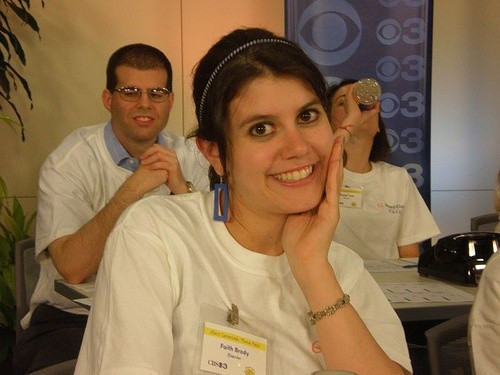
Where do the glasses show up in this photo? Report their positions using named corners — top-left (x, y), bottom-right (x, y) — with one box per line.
top-left (113, 86), bottom-right (171, 103)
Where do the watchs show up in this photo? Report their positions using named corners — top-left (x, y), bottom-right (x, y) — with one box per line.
top-left (170, 181), bottom-right (196, 195)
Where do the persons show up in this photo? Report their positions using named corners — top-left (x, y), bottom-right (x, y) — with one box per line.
top-left (20, 44), bottom-right (211, 375)
top-left (326, 78), bottom-right (441, 261)
top-left (75, 28), bottom-right (412, 375)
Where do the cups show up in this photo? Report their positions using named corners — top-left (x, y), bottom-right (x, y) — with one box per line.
top-left (352, 77), bottom-right (382, 110)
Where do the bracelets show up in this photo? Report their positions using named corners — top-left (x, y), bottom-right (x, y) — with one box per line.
top-left (338, 125), bottom-right (350, 134)
top-left (305, 293), bottom-right (351, 325)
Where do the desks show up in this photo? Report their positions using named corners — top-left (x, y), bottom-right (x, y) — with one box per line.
top-left (53, 257), bottom-right (475, 323)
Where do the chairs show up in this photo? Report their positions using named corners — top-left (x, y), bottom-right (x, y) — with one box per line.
top-left (424, 313), bottom-right (471, 375)
top-left (14, 238), bottom-right (35, 325)
